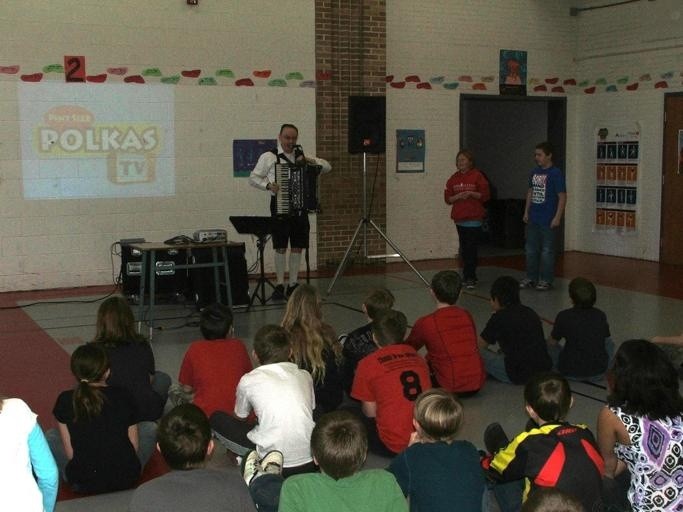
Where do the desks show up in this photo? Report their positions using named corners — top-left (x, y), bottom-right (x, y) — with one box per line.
top-left (129, 240), bottom-right (243, 341)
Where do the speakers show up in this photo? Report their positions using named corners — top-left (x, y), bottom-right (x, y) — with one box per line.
top-left (347, 94), bottom-right (387, 154)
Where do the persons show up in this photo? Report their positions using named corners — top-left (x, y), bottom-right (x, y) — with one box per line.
top-left (0, 272), bottom-right (683, 512)
top-left (518, 142), bottom-right (567, 292)
top-left (249, 124), bottom-right (332, 301)
top-left (444, 151), bottom-right (492, 285)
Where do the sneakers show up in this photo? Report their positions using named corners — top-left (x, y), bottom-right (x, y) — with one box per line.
top-left (238, 447), bottom-right (265, 486)
top-left (482, 421), bottom-right (510, 454)
top-left (519, 277), bottom-right (535, 290)
top-left (287, 283), bottom-right (300, 297)
top-left (272, 284), bottom-right (285, 301)
top-left (535, 280), bottom-right (553, 291)
top-left (259, 448), bottom-right (285, 476)
top-left (462, 274), bottom-right (479, 289)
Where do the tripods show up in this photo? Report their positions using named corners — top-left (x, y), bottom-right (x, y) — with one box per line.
top-left (327, 154), bottom-right (431, 299)
top-left (244, 243), bottom-right (280, 313)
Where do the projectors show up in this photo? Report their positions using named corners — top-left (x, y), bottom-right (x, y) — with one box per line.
top-left (193, 228), bottom-right (228, 242)
